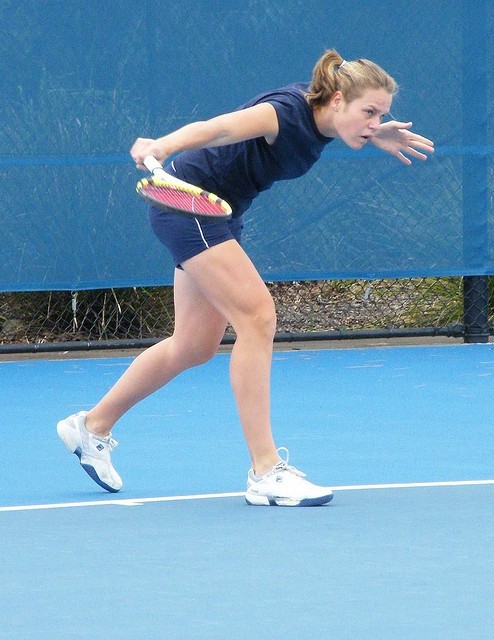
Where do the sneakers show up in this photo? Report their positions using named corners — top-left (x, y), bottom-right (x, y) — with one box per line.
top-left (56, 411), bottom-right (122, 492)
top-left (245, 447), bottom-right (334, 508)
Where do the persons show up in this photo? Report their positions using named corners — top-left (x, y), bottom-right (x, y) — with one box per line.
top-left (55, 47), bottom-right (435, 508)
